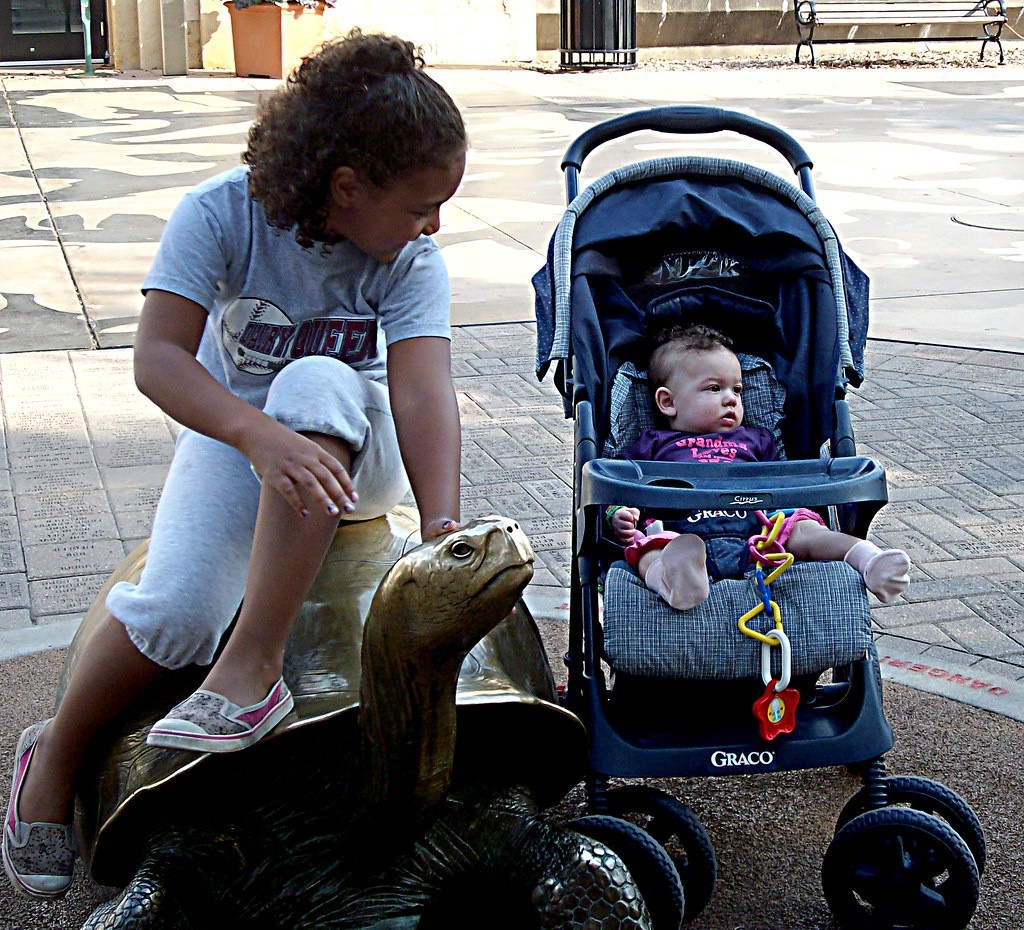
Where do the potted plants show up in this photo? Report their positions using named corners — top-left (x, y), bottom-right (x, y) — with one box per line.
top-left (224, 0), bottom-right (339, 80)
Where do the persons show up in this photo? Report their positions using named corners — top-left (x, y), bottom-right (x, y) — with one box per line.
top-left (603, 326), bottom-right (908, 609)
top-left (2, 33), bottom-right (468, 899)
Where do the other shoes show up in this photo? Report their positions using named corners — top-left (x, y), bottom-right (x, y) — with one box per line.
top-left (1, 717), bottom-right (75, 900)
top-left (146, 677), bottom-right (294, 754)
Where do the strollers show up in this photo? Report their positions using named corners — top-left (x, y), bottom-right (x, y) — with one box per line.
top-left (530, 102), bottom-right (990, 930)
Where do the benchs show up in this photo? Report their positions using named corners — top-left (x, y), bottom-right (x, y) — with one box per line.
top-left (794, 0), bottom-right (1009, 67)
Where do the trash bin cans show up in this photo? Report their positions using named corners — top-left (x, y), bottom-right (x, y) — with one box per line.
top-left (222, 1), bottom-right (338, 80)
top-left (559, 0), bottom-right (640, 71)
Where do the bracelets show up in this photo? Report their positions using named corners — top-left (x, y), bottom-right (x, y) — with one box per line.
top-left (606, 506), bottom-right (622, 524)
